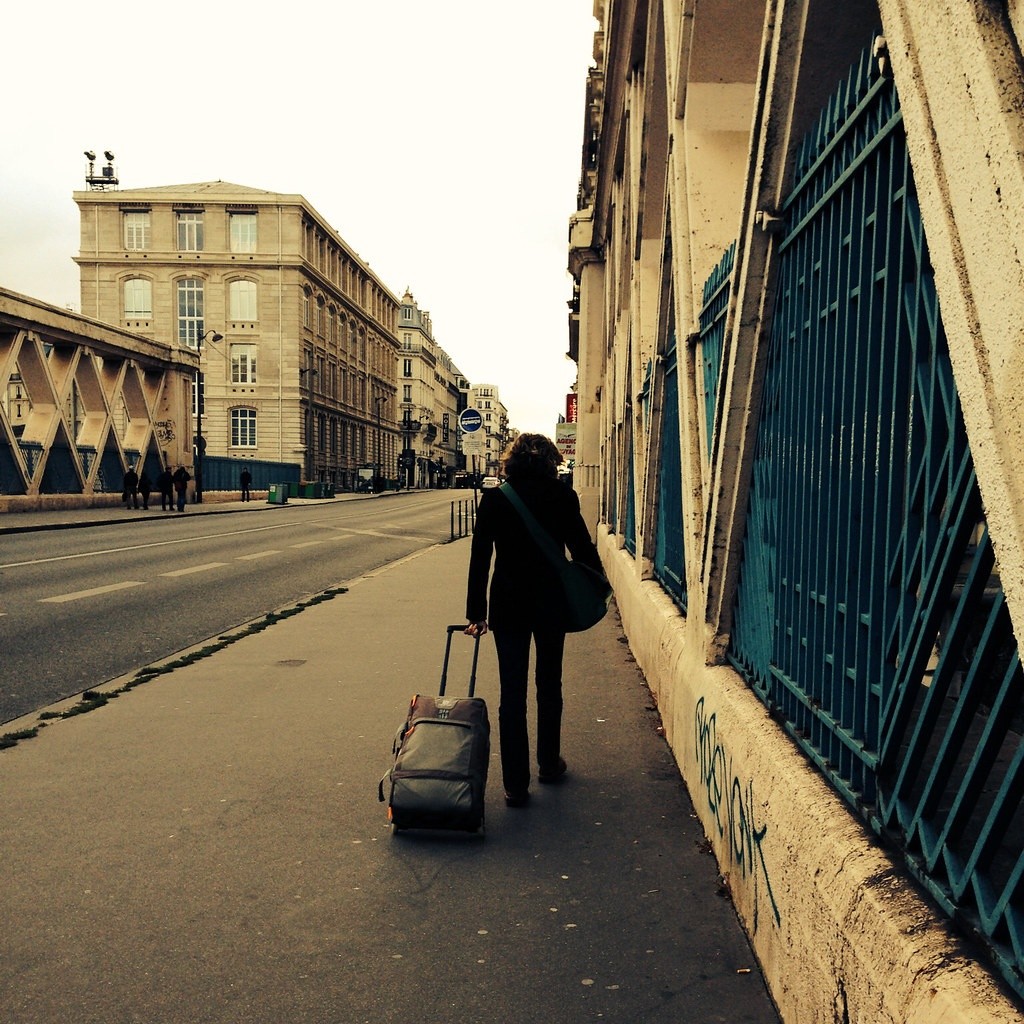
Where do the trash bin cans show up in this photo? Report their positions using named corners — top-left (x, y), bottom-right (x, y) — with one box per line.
top-left (266, 483), bottom-right (288, 504)
top-left (288, 482), bottom-right (298, 498)
top-left (298, 481), bottom-right (335, 498)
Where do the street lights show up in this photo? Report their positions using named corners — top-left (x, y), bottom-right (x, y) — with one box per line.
top-left (377, 397), bottom-right (388, 476)
top-left (195, 323), bottom-right (223, 503)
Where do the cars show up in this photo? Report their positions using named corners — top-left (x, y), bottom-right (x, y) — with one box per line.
top-left (481, 477), bottom-right (501, 491)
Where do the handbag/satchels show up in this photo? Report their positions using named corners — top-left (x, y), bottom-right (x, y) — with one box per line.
top-left (555, 560), bottom-right (609, 634)
top-left (122, 493), bottom-right (127, 502)
top-left (174, 481), bottom-right (183, 492)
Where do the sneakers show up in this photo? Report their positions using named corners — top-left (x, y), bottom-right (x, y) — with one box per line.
top-left (504, 786), bottom-right (531, 807)
top-left (537, 756), bottom-right (567, 784)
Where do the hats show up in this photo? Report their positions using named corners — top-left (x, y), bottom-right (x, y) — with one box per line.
top-left (129, 465), bottom-right (135, 470)
top-left (165, 466), bottom-right (172, 470)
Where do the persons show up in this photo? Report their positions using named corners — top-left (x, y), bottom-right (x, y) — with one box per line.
top-left (465, 435), bottom-right (613, 810)
top-left (122, 463), bottom-right (187, 513)
top-left (239, 467), bottom-right (252, 503)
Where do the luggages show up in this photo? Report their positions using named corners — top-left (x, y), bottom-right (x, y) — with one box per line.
top-left (378, 622), bottom-right (492, 841)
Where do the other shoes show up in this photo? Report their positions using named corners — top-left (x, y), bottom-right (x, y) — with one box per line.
top-left (247, 499), bottom-right (249, 502)
top-left (241, 499), bottom-right (244, 502)
